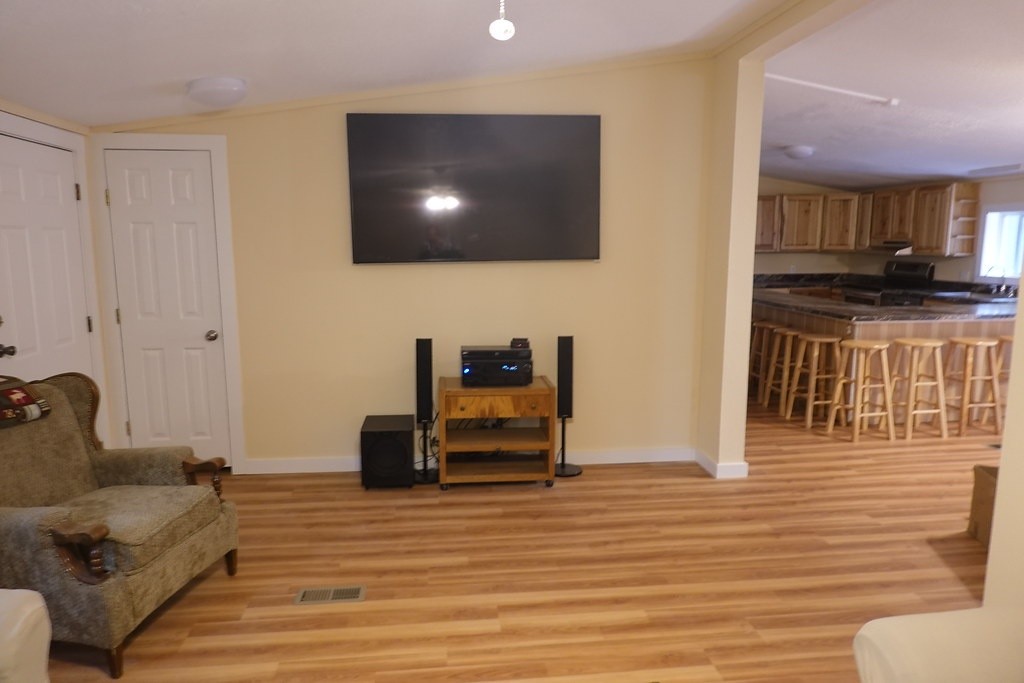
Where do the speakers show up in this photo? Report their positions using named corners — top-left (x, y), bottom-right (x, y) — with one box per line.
top-left (415, 338), bottom-right (440, 483)
top-left (360, 414), bottom-right (415, 490)
top-left (554, 337), bottom-right (582, 477)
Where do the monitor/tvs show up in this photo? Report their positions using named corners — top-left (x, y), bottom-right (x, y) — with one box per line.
top-left (347, 113), bottom-right (601, 264)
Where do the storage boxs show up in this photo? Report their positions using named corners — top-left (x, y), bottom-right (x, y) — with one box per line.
top-left (968, 463), bottom-right (998, 548)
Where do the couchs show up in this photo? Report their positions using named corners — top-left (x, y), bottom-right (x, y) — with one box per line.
top-left (0, 372), bottom-right (238, 683)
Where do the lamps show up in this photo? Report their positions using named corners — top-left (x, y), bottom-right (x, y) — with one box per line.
top-left (488, 0), bottom-right (515, 40)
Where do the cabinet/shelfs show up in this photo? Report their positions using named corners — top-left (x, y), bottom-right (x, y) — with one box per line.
top-left (438, 376), bottom-right (556, 491)
top-left (755, 179), bottom-right (980, 257)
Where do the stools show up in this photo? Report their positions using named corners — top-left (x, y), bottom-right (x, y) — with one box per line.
top-left (749, 320), bottom-right (784, 403)
top-left (786, 333), bottom-right (847, 429)
top-left (826, 339), bottom-right (897, 443)
top-left (981, 335), bottom-right (1013, 425)
top-left (764, 328), bottom-right (811, 417)
top-left (875, 337), bottom-right (950, 438)
top-left (930, 335), bottom-right (1002, 436)
